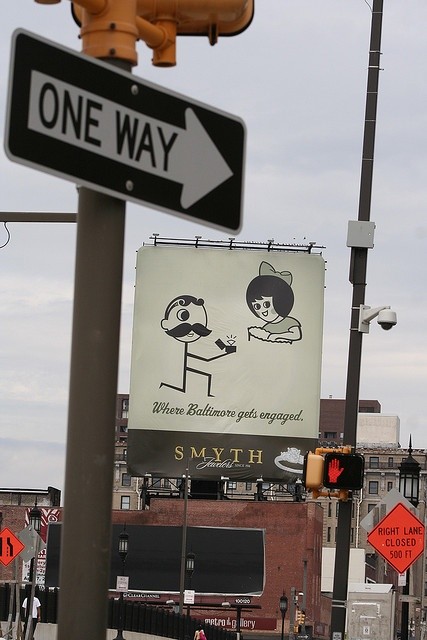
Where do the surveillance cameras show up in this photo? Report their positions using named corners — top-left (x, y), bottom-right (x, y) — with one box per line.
top-left (376, 309), bottom-right (400, 331)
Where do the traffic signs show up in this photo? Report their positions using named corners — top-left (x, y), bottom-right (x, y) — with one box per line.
top-left (5, 24), bottom-right (245, 239)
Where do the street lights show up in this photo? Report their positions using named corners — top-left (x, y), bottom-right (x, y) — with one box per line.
top-left (278, 588), bottom-right (288, 640)
top-left (112, 521), bottom-right (130, 639)
top-left (185, 544), bottom-right (195, 640)
top-left (26, 494), bottom-right (42, 640)
top-left (388, 435), bottom-right (422, 640)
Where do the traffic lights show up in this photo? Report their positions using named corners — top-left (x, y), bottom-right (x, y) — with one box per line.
top-left (321, 451), bottom-right (366, 490)
top-left (302, 450), bottom-right (324, 492)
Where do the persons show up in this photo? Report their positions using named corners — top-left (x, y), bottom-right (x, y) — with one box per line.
top-left (193, 625), bottom-right (207, 639)
top-left (22, 592), bottom-right (41, 640)
